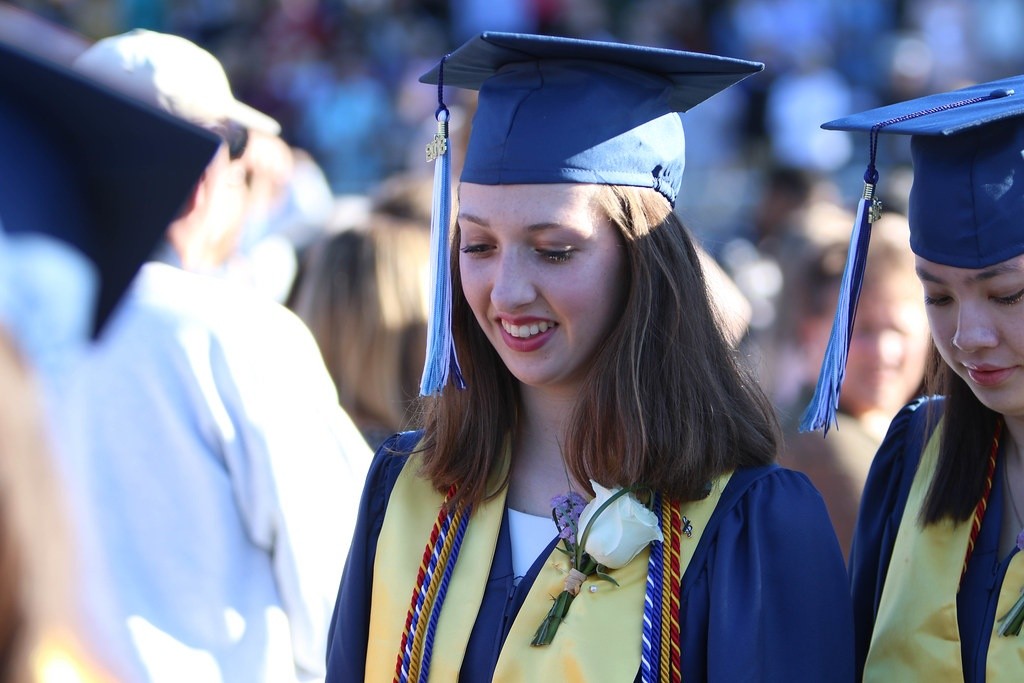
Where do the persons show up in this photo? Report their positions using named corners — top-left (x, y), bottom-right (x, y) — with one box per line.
top-left (324, 30), bottom-right (853, 683)
top-left (0, 1), bottom-right (1024, 683)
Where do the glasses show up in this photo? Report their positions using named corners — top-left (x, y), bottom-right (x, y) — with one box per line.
top-left (215, 122), bottom-right (247, 159)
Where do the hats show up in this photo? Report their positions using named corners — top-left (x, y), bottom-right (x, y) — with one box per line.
top-left (75, 27), bottom-right (281, 138)
top-left (1, 16), bottom-right (213, 341)
top-left (418, 31), bottom-right (765, 397)
top-left (795, 75), bottom-right (1024, 439)
